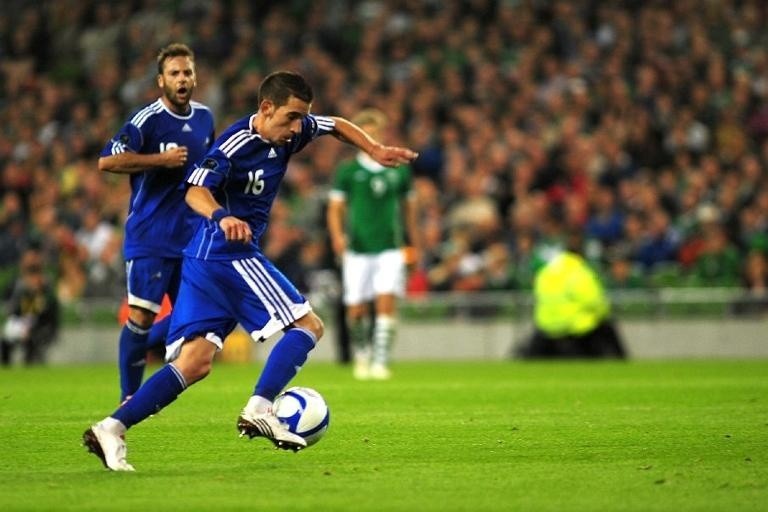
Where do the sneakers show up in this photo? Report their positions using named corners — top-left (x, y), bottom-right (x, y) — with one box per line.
top-left (236, 408), bottom-right (307, 453)
top-left (83, 422), bottom-right (136, 472)
top-left (353, 362), bottom-right (390, 380)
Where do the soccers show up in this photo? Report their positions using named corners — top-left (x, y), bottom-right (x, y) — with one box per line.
top-left (273, 386), bottom-right (330, 448)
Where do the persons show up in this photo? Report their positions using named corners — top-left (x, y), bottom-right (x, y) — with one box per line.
top-left (329, 109), bottom-right (418, 381)
top-left (1, 0), bottom-right (768, 368)
top-left (79, 43), bottom-right (420, 473)
top-left (98, 43), bottom-right (215, 417)
